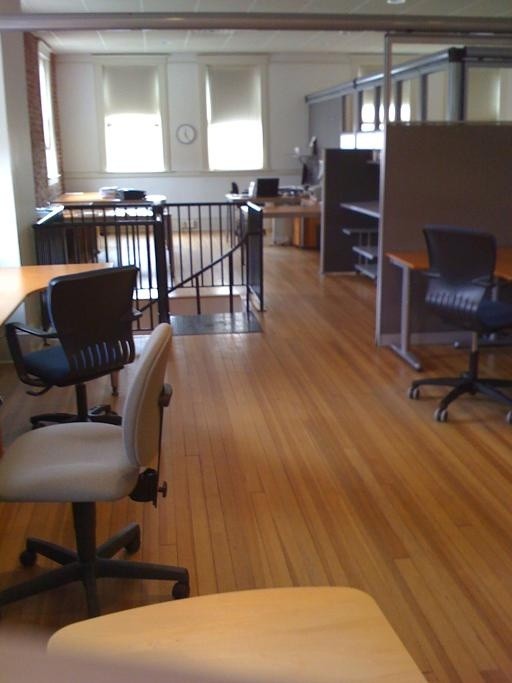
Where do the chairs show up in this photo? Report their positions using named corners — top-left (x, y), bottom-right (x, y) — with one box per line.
top-left (0, 322), bottom-right (191, 618)
top-left (47, 584), bottom-right (428, 683)
top-left (5, 265), bottom-right (139, 429)
top-left (408, 228), bottom-right (512, 425)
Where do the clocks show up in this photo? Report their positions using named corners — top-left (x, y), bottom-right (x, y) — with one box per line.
top-left (176, 124), bottom-right (196, 145)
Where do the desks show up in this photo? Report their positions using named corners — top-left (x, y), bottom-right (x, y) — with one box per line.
top-left (49, 192), bottom-right (175, 278)
top-left (226, 193), bottom-right (310, 248)
top-left (384, 245), bottom-right (512, 371)
top-left (240, 205), bottom-right (321, 249)
top-left (340, 201), bottom-right (380, 281)
top-left (0, 263), bottom-right (114, 328)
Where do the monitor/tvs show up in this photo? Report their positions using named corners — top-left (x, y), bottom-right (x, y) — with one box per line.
top-left (257, 178), bottom-right (279, 198)
